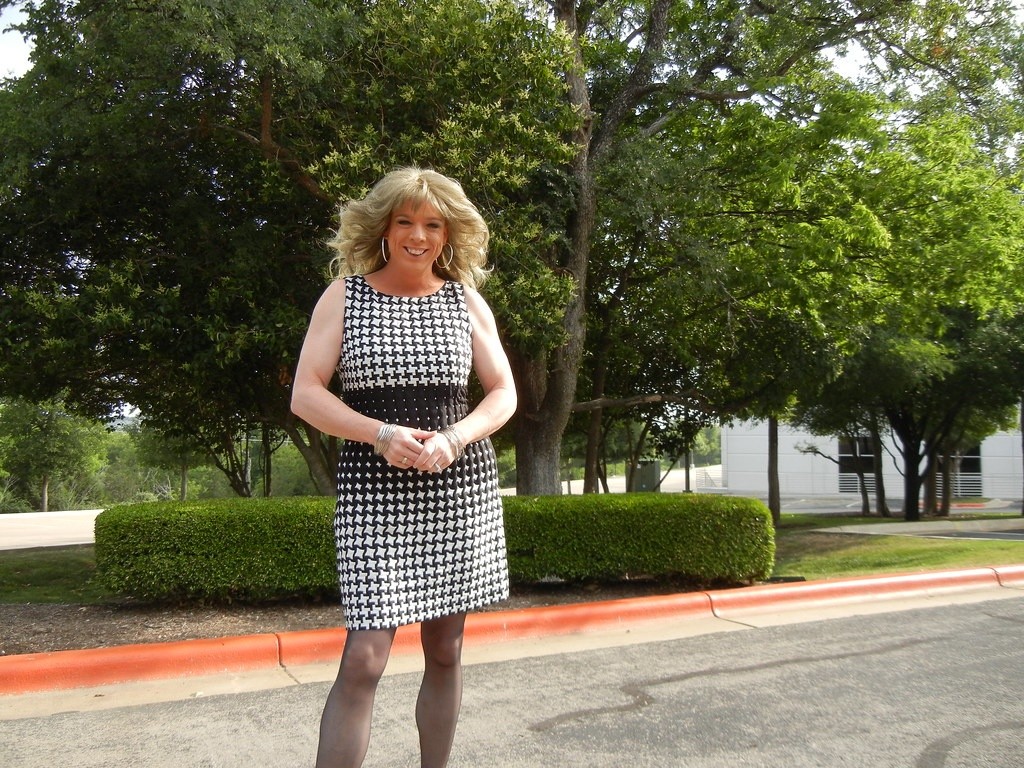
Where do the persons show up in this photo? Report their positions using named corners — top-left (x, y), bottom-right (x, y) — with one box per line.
top-left (290, 169), bottom-right (518, 767)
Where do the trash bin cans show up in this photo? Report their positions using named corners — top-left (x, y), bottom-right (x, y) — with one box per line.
top-left (625, 460), bottom-right (660, 493)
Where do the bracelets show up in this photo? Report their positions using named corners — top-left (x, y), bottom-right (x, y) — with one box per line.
top-left (374, 423), bottom-right (397, 457)
top-left (438, 426), bottom-right (464, 460)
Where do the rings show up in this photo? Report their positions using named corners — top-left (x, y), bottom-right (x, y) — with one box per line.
top-left (435, 463), bottom-right (441, 472)
top-left (402, 457), bottom-right (407, 464)
